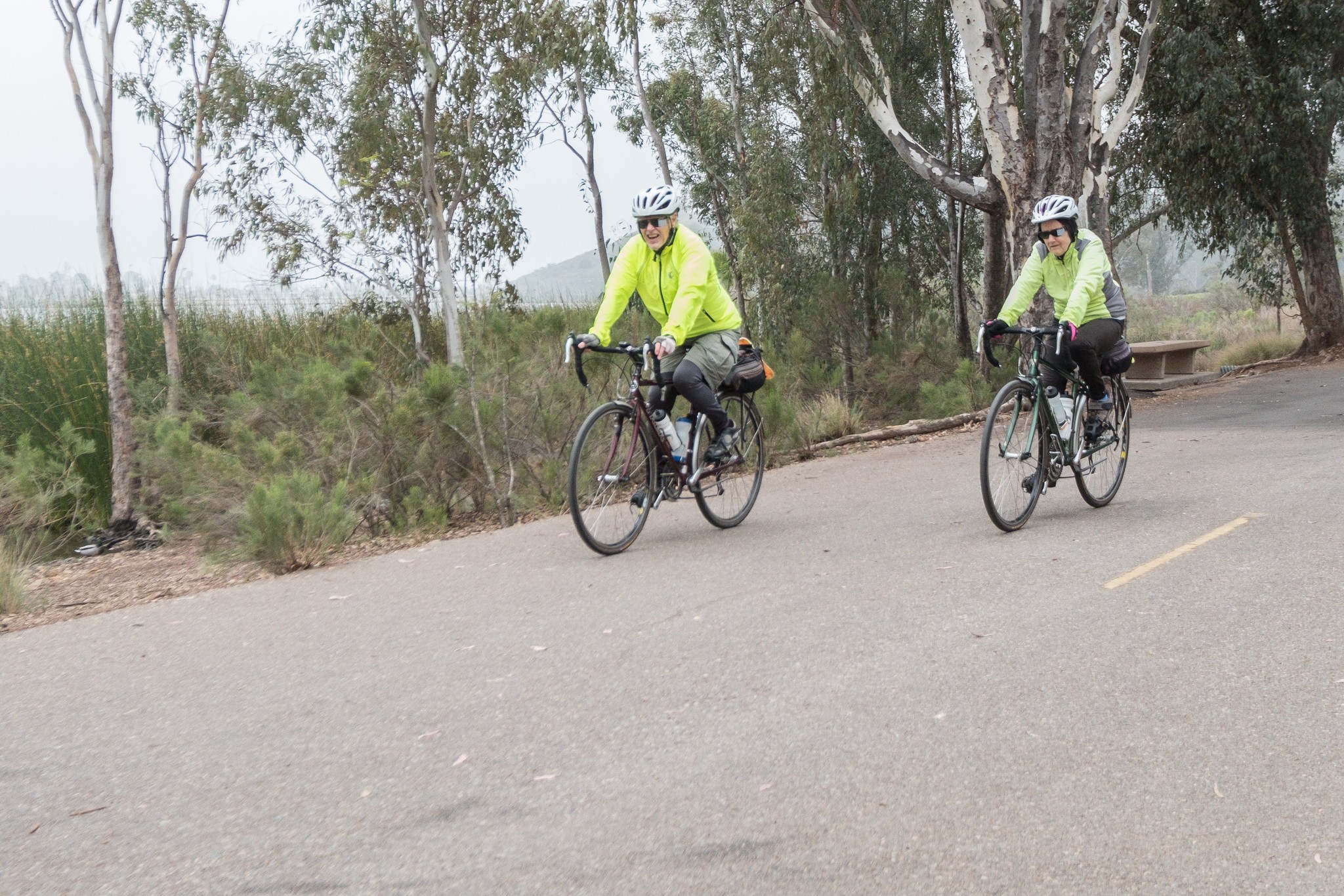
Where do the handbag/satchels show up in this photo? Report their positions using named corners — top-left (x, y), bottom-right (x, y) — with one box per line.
top-left (721, 347), bottom-right (767, 394)
top-left (1098, 334), bottom-right (1134, 376)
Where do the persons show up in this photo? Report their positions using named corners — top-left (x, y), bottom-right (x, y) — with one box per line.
top-left (986, 194), bottom-right (1127, 490)
top-left (574, 183), bottom-right (742, 508)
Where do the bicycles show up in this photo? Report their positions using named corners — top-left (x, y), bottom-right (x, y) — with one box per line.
top-left (979, 323), bottom-right (1132, 532)
top-left (568, 327), bottom-right (765, 555)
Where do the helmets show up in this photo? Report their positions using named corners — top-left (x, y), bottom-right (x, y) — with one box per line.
top-left (1030, 194), bottom-right (1079, 225)
top-left (631, 184), bottom-right (680, 218)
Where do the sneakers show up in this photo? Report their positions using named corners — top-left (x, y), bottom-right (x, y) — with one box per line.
top-left (703, 418), bottom-right (741, 463)
top-left (1020, 463), bottom-right (1057, 493)
top-left (629, 484), bottom-right (676, 507)
top-left (1083, 388), bottom-right (1113, 443)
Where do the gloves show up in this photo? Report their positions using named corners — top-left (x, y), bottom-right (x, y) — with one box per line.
top-left (1059, 320), bottom-right (1078, 344)
top-left (985, 318), bottom-right (1008, 341)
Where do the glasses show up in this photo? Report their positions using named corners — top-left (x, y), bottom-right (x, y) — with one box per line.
top-left (1037, 225), bottom-right (1067, 239)
top-left (637, 217), bottom-right (670, 230)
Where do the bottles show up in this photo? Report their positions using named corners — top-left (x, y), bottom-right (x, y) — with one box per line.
top-left (1045, 386), bottom-right (1068, 428)
top-left (1055, 390), bottom-right (1073, 441)
top-left (670, 413), bottom-right (693, 464)
top-left (653, 409), bottom-right (684, 451)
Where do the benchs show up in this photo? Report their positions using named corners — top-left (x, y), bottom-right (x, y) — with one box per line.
top-left (1123, 339), bottom-right (1211, 380)
